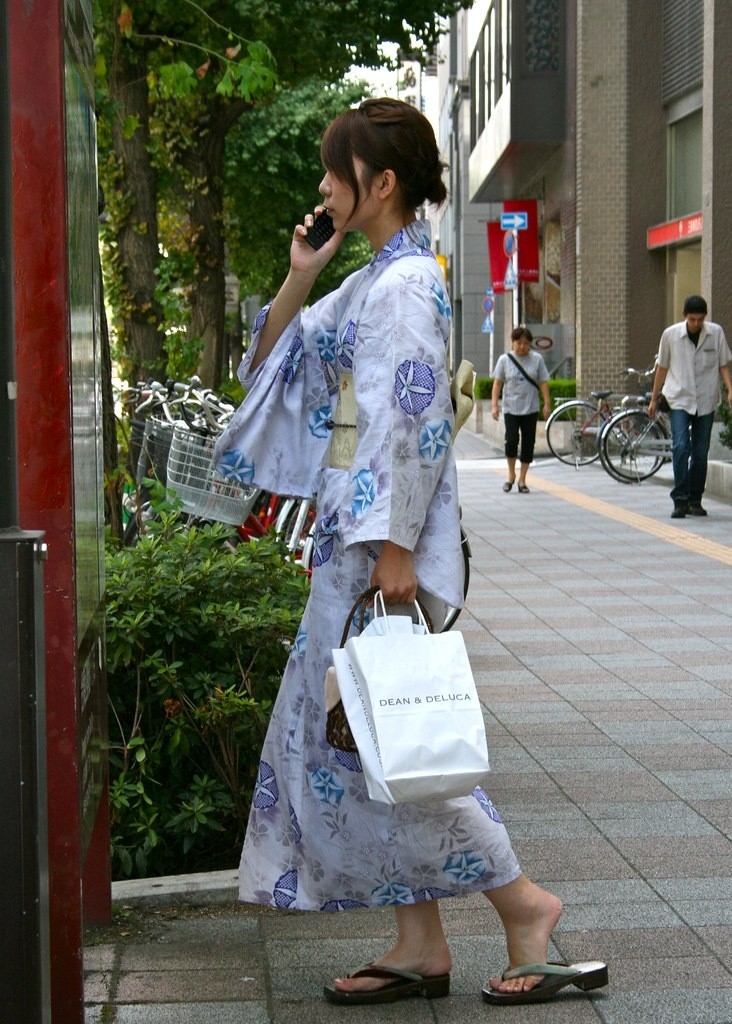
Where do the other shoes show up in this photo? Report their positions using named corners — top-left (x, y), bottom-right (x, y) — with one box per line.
top-left (688, 504), bottom-right (707, 516)
top-left (670, 501), bottom-right (687, 519)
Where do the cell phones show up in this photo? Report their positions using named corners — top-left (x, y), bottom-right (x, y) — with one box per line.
top-left (302, 208), bottom-right (336, 251)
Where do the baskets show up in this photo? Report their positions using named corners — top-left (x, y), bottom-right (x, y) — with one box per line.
top-left (136, 419), bottom-right (176, 498)
top-left (127, 419), bottom-right (145, 481)
top-left (166, 427), bottom-right (262, 525)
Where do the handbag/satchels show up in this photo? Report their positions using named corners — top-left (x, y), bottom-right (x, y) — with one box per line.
top-left (330, 591), bottom-right (493, 804)
top-left (325, 584), bottom-right (433, 754)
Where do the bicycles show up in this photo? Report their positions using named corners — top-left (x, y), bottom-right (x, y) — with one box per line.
top-left (544, 353), bottom-right (661, 472)
top-left (597, 393), bottom-right (692, 490)
top-left (123, 374), bottom-right (473, 633)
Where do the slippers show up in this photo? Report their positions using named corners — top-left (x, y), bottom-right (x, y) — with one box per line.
top-left (518, 483), bottom-right (529, 493)
top-left (325, 966), bottom-right (450, 1002)
top-left (503, 478), bottom-right (515, 492)
top-left (482, 960), bottom-right (608, 1003)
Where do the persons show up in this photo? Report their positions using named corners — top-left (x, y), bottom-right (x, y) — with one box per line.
top-left (210, 98), bottom-right (609, 1006)
top-left (647, 296), bottom-right (732, 519)
top-left (490, 327), bottom-right (553, 494)
